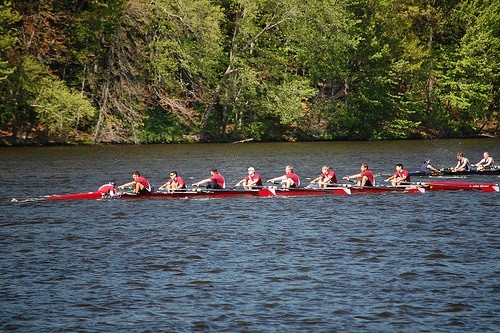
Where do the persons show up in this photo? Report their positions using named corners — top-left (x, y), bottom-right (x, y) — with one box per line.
top-left (475, 151), bottom-right (495, 172)
top-left (342, 162), bottom-right (375, 189)
top-left (192, 167), bottom-right (225, 191)
top-left (97, 181), bottom-right (123, 198)
top-left (448, 152), bottom-right (472, 173)
top-left (233, 167), bottom-right (262, 189)
top-left (266, 166), bottom-right (301, 191)
top-left (119, 170), bottom-right (152, 195)
top-left (158, 171), bottom-right (188, 192)
top-left (383, 164), bottom-right (411, 186)
top-left (421, 158), bottom-right (441, 176)
top-left (310, 166), bottom-right (338, 188)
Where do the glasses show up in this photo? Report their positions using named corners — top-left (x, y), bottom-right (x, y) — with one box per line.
top-left (133, 175), bottom-right (139, 178)
top-left (170, 173), bottom-right (175, 177)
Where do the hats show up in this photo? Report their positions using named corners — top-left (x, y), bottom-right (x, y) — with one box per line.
top-left (109, 180), bottom-right (118, 185)
top-left (322, 167), bottom-right (329, 170)
top-left (425, 159), bottom-right (432, 165)
top-left (286, 165), bottom-right (295, 170)
top-left (248, 167), bottom-right (255, 171)
top-left (209, 167), bottom-right (218, 172)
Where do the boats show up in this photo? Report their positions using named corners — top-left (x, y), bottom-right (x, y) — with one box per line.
top-left (380, 166), bottom-right (500, 177)
top-left (44, 182), bottom-right (499, 200)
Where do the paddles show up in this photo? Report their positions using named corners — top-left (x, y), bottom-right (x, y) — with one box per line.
top-left (118, 179), bottom-right (483, 196)
